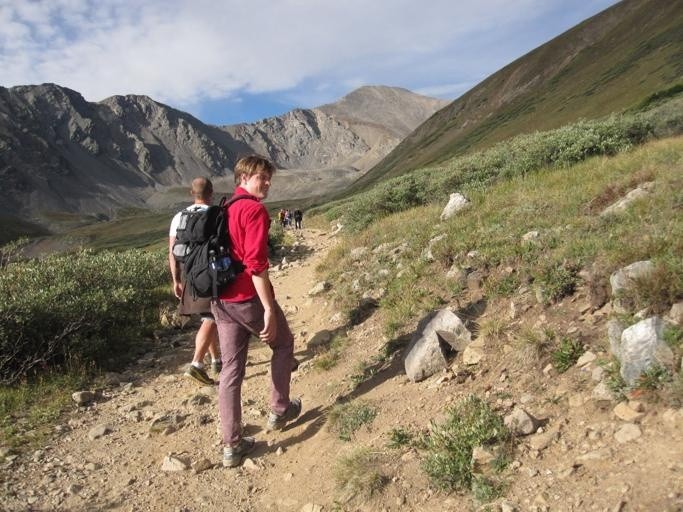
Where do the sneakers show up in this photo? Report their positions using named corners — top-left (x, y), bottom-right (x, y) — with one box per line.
top-left (211, 360), bottom-right (221, 375)
top-left (266, 397), bottom-right (302, 430)
top-left (184, 365), bottom-right (214, 387)
top-left (222, 436), bottom-right (255, 467)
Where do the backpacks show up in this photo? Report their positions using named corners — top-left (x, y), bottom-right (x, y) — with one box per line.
top-left (170, 208), bottom-right (208, 262)
top-left (181, 194), bottom-right (257, 307)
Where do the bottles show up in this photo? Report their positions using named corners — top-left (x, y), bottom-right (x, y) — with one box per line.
top-left (208, 246), bottom-right (235, 283)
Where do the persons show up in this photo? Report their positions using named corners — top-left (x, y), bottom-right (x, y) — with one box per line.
top-left (169, 177), bottom-right (222, 386)
top-left (279, 208), bottom-right (302, 230)
top-left (210, 155), bottom-right (302, 467)
